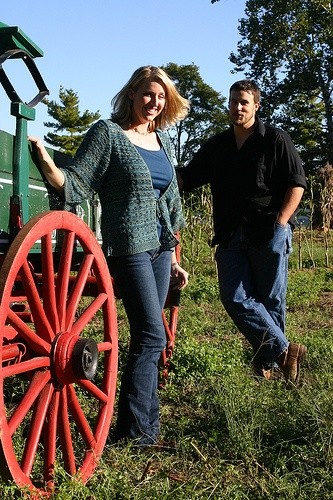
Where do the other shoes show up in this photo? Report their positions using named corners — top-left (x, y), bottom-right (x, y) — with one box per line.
top-left (136, 439), bottom-right (175, 457)
top-left (281, 343), bottom-right (307, 390)
top-left (252, 367), bottom-right (270, 386)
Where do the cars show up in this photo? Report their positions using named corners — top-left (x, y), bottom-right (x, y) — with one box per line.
top-left (287, 209), bottom-right (314, 227)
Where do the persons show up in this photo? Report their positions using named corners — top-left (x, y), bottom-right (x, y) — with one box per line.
top-left (26, 64), bottom-right (189, 447)
top-left (173, 80), bottom-right (307, 390)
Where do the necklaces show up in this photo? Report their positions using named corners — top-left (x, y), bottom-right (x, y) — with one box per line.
top-left (126, 122), bottom-right (150, 135)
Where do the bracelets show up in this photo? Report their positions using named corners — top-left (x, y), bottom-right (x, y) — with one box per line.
top-left (172, 262), bottom-right (177, 266)
